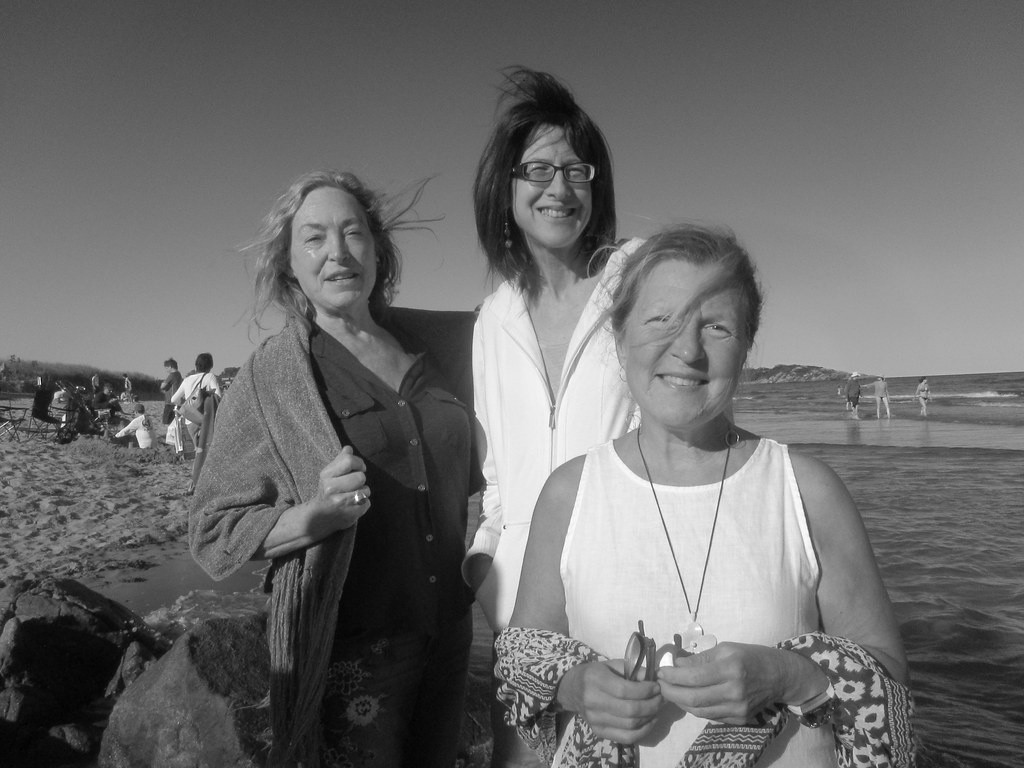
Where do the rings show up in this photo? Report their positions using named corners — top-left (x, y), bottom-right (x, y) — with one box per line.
top-left (354, 492), bottom-right (368, 506)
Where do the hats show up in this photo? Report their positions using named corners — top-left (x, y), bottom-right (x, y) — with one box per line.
top-left (134, 404), bottom-right (144, 410)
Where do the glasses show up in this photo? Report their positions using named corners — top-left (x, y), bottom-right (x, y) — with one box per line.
top-left (511, 161), bottom-right (596, 184)
top-left (623, 620), bottom-right (656, 681)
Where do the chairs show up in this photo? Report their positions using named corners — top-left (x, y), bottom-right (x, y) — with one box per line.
top-left (27, 370), bottom-right (73, 445)
top-left (0, 397), bottom-right (29, 444)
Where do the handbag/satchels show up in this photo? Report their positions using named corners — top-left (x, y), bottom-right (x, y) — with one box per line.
top-left (180, 372), bottom-right (208, 425)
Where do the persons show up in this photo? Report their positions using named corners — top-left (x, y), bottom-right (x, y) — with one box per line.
top-left (510, 223), bottom-right (917, 768)
top-left (470, 68), bottom-right (652, 768)
top-left (91, 354), bottom-right (224, 493)
top-left (193, 168), bottom-right (476, 766)
top-left (841, 372), bottom-right (933, 420)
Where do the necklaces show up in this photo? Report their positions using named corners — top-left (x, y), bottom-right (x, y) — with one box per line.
top-left (636, 413), bottom-right (733, 656)
